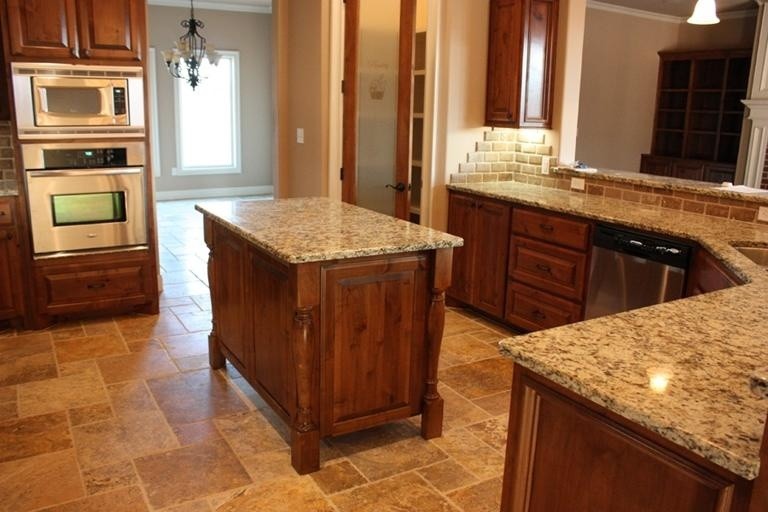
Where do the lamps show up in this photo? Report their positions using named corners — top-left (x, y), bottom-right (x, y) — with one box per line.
top-left (687, 0), bottom-right (721, 25)
top-left (162, 0), bottom-right (221, 90)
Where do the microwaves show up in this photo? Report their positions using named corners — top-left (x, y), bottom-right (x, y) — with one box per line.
top-left (9, 58), bottom-right (146, 137)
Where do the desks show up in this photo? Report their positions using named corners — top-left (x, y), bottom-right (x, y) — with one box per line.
top-left (195, 196), bottom-right (463, 474)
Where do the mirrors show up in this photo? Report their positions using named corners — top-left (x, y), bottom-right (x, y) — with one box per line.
top-left (559, 0), bottom-right (768, 188)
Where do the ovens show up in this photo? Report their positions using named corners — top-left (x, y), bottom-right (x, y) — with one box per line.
top-left (20, 142), bottom-right (155, 256)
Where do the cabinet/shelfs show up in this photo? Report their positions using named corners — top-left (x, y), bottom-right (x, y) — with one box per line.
top-left (640, 48), bottom-right (752, 184)
top-left (0, 1), bottom-right (146, 66)
top-left (31, 249), bottom-right (159, 330)
top-left (448, 191), bottom-right (592, 333)
top-left (0, 195), bottom-right (31, 329)
top-left (686, 249), bottom-right (744, 301)
top-left (485, 0), bottom-right (560, 128)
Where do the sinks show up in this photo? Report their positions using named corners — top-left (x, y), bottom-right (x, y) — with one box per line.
top-left (728, 240), bottom-right (768, 267)
